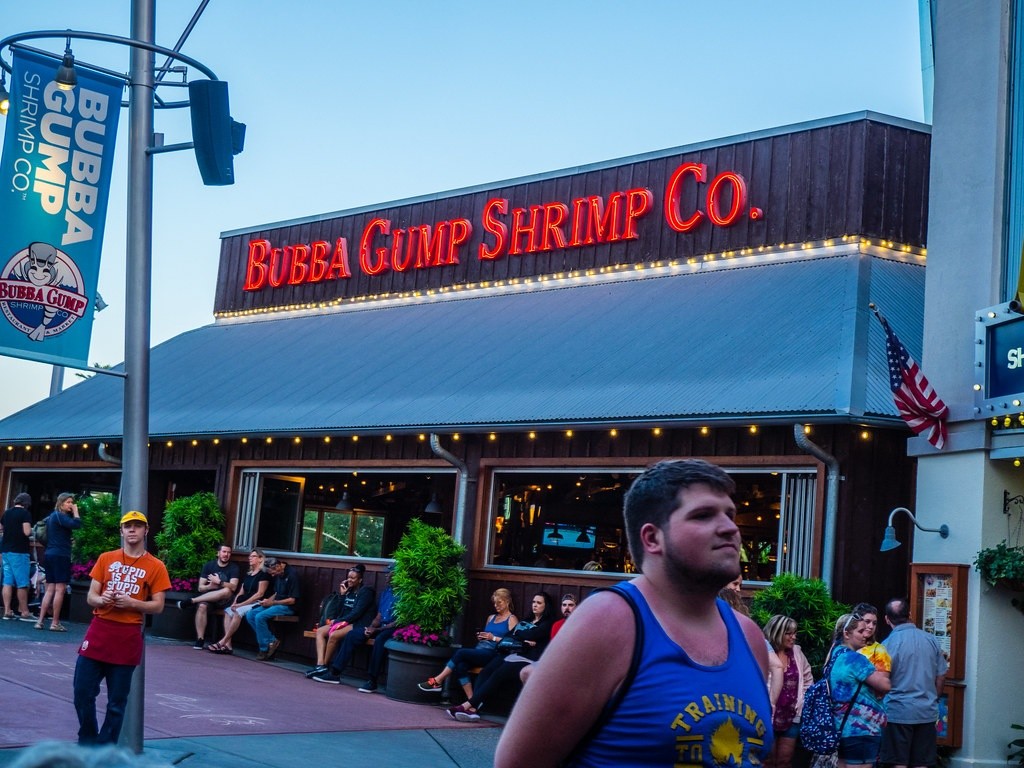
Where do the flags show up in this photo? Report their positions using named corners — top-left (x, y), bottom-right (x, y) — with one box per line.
top-left (882, 317), bottom-right (950, 450)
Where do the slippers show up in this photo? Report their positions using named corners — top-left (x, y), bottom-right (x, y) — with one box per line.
top-left (207, 642), bottom-right (221, 650)
top-left (214, 645), bottom-right (233, 654)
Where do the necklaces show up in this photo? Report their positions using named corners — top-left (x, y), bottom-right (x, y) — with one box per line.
top-left (122, 546), bottom-right (146, 573)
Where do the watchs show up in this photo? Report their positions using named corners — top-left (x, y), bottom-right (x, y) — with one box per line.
top-left (219, 579), bottom-right (224, 585)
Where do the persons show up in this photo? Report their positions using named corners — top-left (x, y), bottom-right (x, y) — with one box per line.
top-left (73, 510), bottom-right (173, 746)
top-left (493, 457), bottom-right (772, 768)
top-left (32, 492), bottom-right (81, 631)
top-left (418, 587), bottom-right (577, 722)
top-left (176, 543), bottom-right (397, 695)
top-left (720, 572), bottom-right (947, 768)
top-left (0, 493), bottom-right (39, 623)
top-left (245, 558), bottom-right (302, 661)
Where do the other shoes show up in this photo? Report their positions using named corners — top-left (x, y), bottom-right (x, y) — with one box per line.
top-left (456, 711), bottom-right (480, 722)
top-left (257, 652), bottom-right (268, 660)
top-left (267, 639), bottom-right (281, 658)
top-left (305, 665), bottom-right (328, 677)
top-left (446, 705), bottom-right (464, 720)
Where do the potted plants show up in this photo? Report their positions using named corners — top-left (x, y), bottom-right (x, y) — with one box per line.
top-left (973, 539), bottom-right (1024, 594)
top-left (384, 517), bottom-right (470, 708)
top-left (151, 491), bottom-right (226, 642)
top-left (66, 494), bottom-right (122, 622)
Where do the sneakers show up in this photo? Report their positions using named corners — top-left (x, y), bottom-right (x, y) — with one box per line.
top-left (20, 613), bottom-right (38, 622)
top-left (3, 611), bottom-right (17, 620)
top-left (312, 672), bottom-right (340, 684)
top-left (178, 598), bottom-right (192, 609)
top-left (359, 680), bottom-right (377, 692)
top-left (417, 677), bottom-right (442, 692)
top-left (194, 638), bottom-right (204, 650)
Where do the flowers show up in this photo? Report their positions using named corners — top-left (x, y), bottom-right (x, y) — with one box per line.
top-left (167, 577), bottom-right (201, 593)
top-left (70, 559), bottom-right (97, 580)
top-left (392, 623), bottom-right (453, 647)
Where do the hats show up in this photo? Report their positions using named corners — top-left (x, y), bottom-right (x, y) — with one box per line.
top-left (120, 511), bottom-right (147, 527)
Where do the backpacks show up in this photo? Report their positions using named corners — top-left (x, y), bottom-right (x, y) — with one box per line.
top-left (799, 647), bottom-right (862, 754)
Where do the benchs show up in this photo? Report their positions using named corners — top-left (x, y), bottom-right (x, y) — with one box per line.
top-left (304, 629), bottom-right (376, 666)
top-left (206, 607), bottom-right (300, 644)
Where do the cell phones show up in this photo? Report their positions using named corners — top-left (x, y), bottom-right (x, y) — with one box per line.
top-left (343, 580), bottom-right (348, 589)
top-left (257, 600), bottom-right (263, 603)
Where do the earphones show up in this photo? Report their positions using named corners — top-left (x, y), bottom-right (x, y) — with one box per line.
top-left (144, 529), bottom-right (148, 536)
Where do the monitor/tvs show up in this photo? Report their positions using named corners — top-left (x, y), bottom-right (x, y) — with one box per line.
top-left (540, 520), bottom-right (597, 550)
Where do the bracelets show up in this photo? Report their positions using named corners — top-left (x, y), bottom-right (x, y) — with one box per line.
top-left (369, 624), bottom-right (376, 628)
top-left (492, 635), bottom-right (496, 642)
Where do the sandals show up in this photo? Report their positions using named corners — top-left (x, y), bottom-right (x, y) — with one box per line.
top-left (50, 624), bottom-right (68, 631)
top-left (34, 623), bottom-right (43, 629)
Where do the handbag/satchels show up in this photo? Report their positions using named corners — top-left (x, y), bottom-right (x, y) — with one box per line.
top-left (496, 620), bottom-right (538, 650)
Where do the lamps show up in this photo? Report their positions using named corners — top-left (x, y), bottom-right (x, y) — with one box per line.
top-left (547, 523), bottom-right (564, 539)
top-left (879, 507), bottom-right (950, 551)
top-left (575, 528), bottom-right (590, 542)
top-left (423, 475), bottom-right (444, 514)
top-left (334, 474), bottom-right (353, 511)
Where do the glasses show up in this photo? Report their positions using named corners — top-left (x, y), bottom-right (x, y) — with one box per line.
top-left (249, 555), bottom-right (259, 558)
top-left (843, 614), bottom-right (863, 632)
top-left (860, 604), bottom-right (877, 612)
top-left (786, 631), bottom-right (797, 637)
